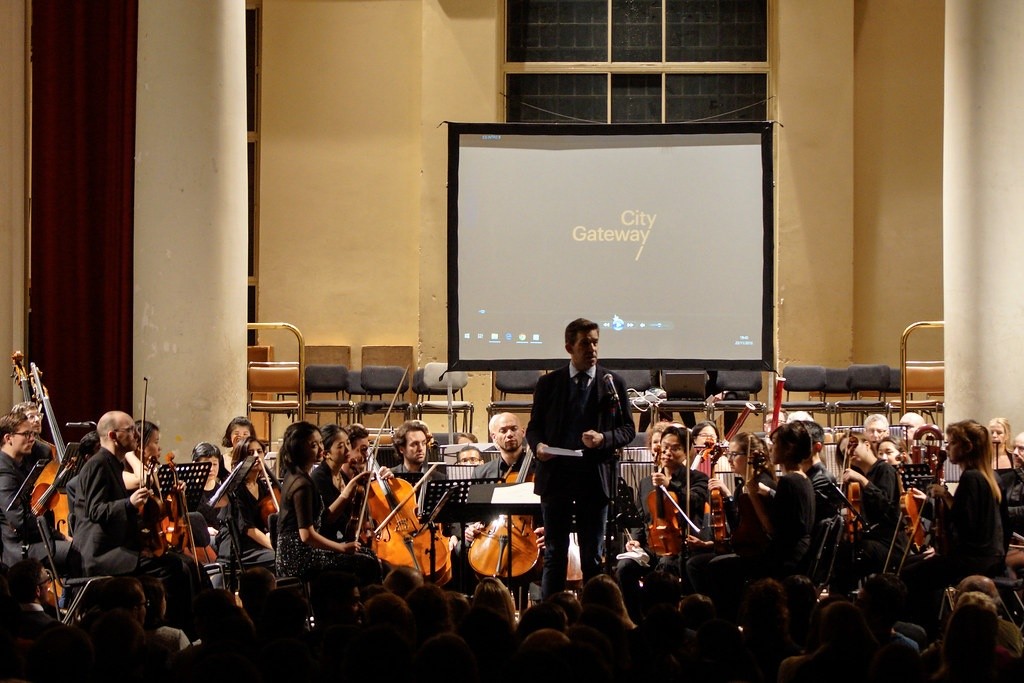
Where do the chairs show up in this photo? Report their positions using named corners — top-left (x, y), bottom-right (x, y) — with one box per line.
top-left (247, 361), bottom-right (948, 435)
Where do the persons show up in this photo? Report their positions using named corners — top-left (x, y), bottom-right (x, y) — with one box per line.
top-left (523, 318), bottom-right (637, 605)
top-left (0, 381), bottom-right (1024, 683)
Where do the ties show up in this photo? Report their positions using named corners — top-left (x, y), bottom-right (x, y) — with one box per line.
top-left (572, 371), bottom-right (590, 419)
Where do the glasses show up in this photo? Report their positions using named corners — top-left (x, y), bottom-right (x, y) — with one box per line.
top-left (36, 575), bottom-right (53, 588)
top-left (696, 433), bottom-right (717, 439)
top-left (26, 413), bottom-right (44, 421)
top-left (135, 599), bottom-right (151, 610)
top-left (723, 453), bottom-right (748, 459)
top-left (12, 431), bottom-right (35, 440)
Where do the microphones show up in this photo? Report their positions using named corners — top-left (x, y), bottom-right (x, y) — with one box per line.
top-left (603, 374), bottom-right (620, 404)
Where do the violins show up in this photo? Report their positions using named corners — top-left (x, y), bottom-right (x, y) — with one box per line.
top-left (166, 451), bottom-right (189, 553)
top-left (844, 438), bottom-right (863, 543)
top-left (708, 443), bottom-right (727, 542)
top-left (730, 449), bottom-right (769, 551)
top-left (342, 456), bottom-right (367, 544)
top-left (136, 456), bottom-right (167, 558)
top-left (895, 468), bottom-right (927, 554)
top-left (29, 455), bottom-right (77, 520)
top-left (646, 446), bottom-right (687, 558)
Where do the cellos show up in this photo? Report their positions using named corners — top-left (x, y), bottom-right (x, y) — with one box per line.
top-left (260, 459), bottom-right (282, 528)
top-left (11, 350), bottom-right (56, 459)
top-left (409, 432), bottom-right (453, 587)
top-left (467, 448), bottom-right (542, 579)
top-left (365, 447), bottom-right (448, 579)
top-left (28, 359), bottom-right (75, 609)
